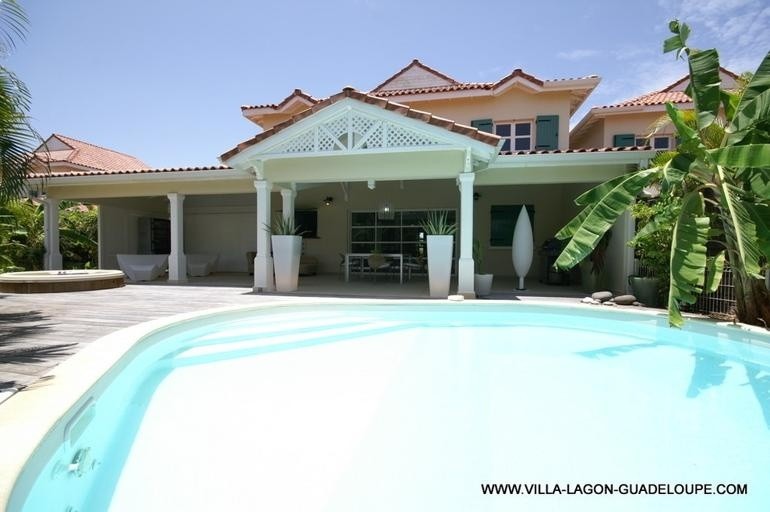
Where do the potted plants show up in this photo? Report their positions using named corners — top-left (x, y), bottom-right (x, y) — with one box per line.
top-left (418, 207), bottom-right (462, 298)
top-left (259, 209), bottom-right (317, 292)
top-left (472, 237), bottom-right (496, 298)
top-left (623, 201), bottom-right (667, 310)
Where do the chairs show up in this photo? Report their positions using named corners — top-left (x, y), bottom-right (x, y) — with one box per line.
top-left (244, 250), bottom-right (257, 275)
top-left (113, 250), bottom-right (221, 284)
top-left (336, 248), bottom-right (429, 284)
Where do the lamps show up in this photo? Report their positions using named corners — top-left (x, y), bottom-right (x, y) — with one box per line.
top-left (322, 196), bottom-right (334, 208)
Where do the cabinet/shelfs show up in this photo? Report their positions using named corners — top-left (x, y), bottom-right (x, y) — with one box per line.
top-left (135, 213), bottom-right (172, 255)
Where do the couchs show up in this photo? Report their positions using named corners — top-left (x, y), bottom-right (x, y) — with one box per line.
top-left (297, 254), bottom-right (319, 276)
